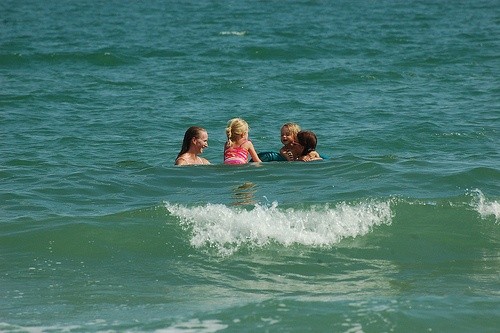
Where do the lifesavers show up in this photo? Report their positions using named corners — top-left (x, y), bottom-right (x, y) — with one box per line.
top-left (250, 151), bottom-right (286, 164)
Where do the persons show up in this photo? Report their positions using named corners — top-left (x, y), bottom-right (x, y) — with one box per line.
top-left (291, 130), bottom-right (324, 162)
top-left (174, 126), bottom-right (213, 166)
top-left (223, 117), bottom-right (263, 163)
top-left (279, 122), bottom-right (320, 162)
